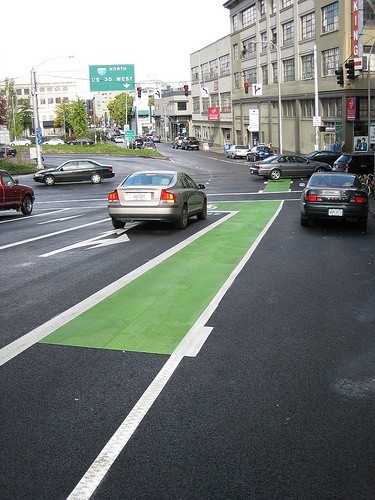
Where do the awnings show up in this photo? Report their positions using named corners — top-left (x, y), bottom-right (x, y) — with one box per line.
top-left (138, 110), bottom-right (153, 116)
top-left (129, 111), bottom-right (135, 116)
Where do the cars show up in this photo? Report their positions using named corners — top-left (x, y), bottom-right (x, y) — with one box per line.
top-left (106, 128), bottom-right (200, 151)
top-left (305, 151), bottom-right (341, 168)
top-left (107, 171), bottom-right (207, 229)
top-left (226, 143), bottom-right (275, 162)
top-left (299, 173), bottom-right (370, 230)
top-left (9, 138), bottom-right (32, 147)
top-left (0, 170), bottom-right (35, 215)
top-left (332, 153), bottom-right (375, 177)
top-left (33, 159), bottom-right (115, 186)
top-left (0, 147), bottom-right (17, 158)
top-left (42, 136), bottom-right (94, 145)
top-left (249, 154), bottom-right (332, 180)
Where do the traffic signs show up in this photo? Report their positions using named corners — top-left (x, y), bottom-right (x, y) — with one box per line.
top-left (89, 64), bottom-right (135, 91)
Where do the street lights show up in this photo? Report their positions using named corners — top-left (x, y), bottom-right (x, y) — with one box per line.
top-left (248, 41), bottom-right (283, 155)
top-left (30, 55), bottom-right (75, 169)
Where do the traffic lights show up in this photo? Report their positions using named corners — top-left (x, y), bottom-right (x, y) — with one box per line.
top-left (345, 60), bottom-right (355, 79)
top-left (335, 69), bottom-right (344, 85)
top-left (137, 87), bottom-right (141, 97)
top-left (244, 83), bottom-right (248, 94)
top-left (184, 85), bottom-right (188, 96)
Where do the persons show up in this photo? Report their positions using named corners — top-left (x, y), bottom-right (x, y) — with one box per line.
top-left (333, 140), bottom-right (347, 153)
top-left (355, 138), bottom-right (367, 151)
